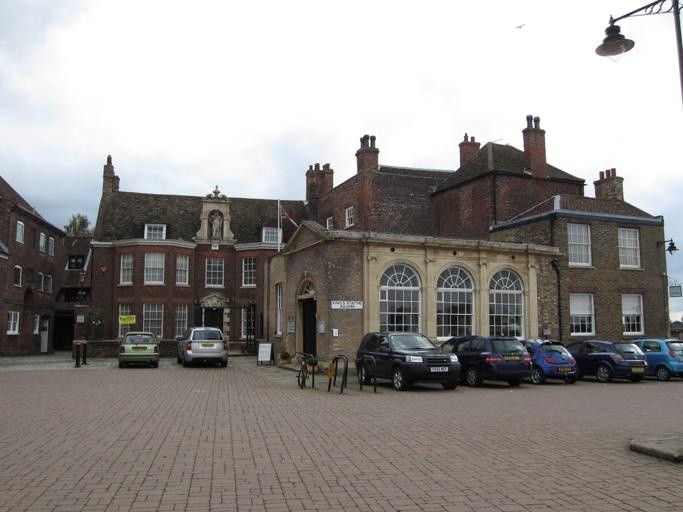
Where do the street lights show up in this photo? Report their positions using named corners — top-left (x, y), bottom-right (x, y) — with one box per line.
top-left (593, 0), bottom-right (683, 100)
top-left (75, 268), bottom-right (89, 307)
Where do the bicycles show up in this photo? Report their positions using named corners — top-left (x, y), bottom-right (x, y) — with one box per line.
top-left (292, 351), bottom-right (315, 388)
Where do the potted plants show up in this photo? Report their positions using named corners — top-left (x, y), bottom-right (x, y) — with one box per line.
top-left (306, 358), bottom-right (319, 372)
top-left (279, 351), bottom-right (289, 364)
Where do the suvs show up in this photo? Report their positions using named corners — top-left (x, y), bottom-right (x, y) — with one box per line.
top-left (351, 330), bottom-right (464, 394)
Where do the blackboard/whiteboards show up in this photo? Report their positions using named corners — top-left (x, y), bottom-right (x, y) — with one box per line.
top-left (257, 342), bottom-right (272, 361)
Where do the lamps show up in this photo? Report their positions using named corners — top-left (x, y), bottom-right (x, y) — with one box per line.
top-left (98, 260), bottom-right (106, 274)
top-left (654, 237), bottom-right (678, 258)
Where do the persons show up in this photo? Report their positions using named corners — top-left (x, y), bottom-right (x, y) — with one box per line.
top-left (208, 210), bottom-right (224, 239)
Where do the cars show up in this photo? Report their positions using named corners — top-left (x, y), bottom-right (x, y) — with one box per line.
top-left (512, 334), bottom-right (580, 387)
top-left (625, 335), bottom-right (683, 384)
top-left (438, 332), bottom-right (534, 387)
top-left (560, 335), bottom-right (649, 385)
top-left (118, 331), bottom-right (161, 368)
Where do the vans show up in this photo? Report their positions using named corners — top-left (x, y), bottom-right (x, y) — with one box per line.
top-left (175, 326), bottom-right (231, 367)
